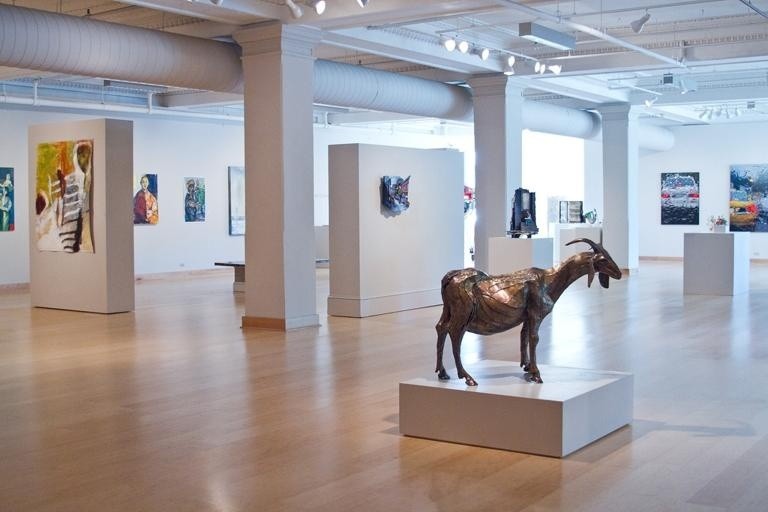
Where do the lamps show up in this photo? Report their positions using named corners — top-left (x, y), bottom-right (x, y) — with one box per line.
top-left (432, 23), bottom-right (576, 78)
top-left (630, 8), bottom-right (653, 35)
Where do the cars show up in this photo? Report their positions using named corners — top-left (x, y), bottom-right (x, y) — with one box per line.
top-left (661, 176), bottom-right (699, 208)
top-left (730, 190), bottom-right (757, 226)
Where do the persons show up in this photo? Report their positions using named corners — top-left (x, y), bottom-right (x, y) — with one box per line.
top-left (185, 179), bottom-right (201, 222)
top-left (1, 187), bottom-right (12, 231)
top-left (0, 173), bottom-right (13, 186)
top-left (134, 176), bottom-right (158, 224)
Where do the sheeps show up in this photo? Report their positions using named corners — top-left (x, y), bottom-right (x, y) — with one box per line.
top-left (434, 229), bottom-right (622, 386)
